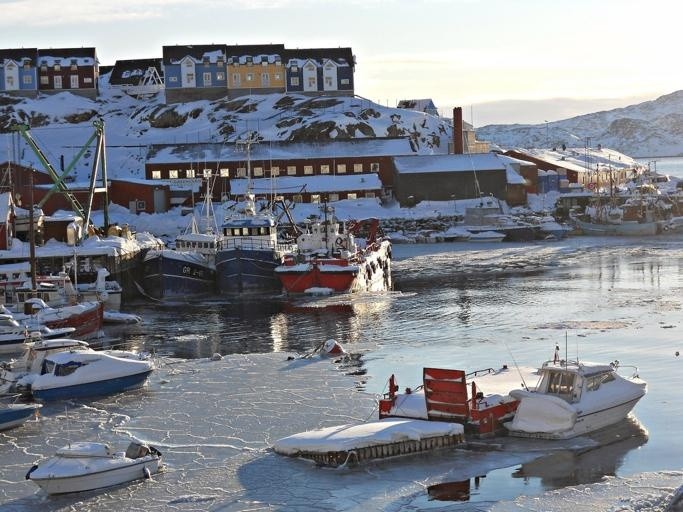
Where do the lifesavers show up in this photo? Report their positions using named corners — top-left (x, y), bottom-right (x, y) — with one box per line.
top-left (335, 237), bottom-right (342, 245)
top-left (589, 183), bottom-right (595, 190)
